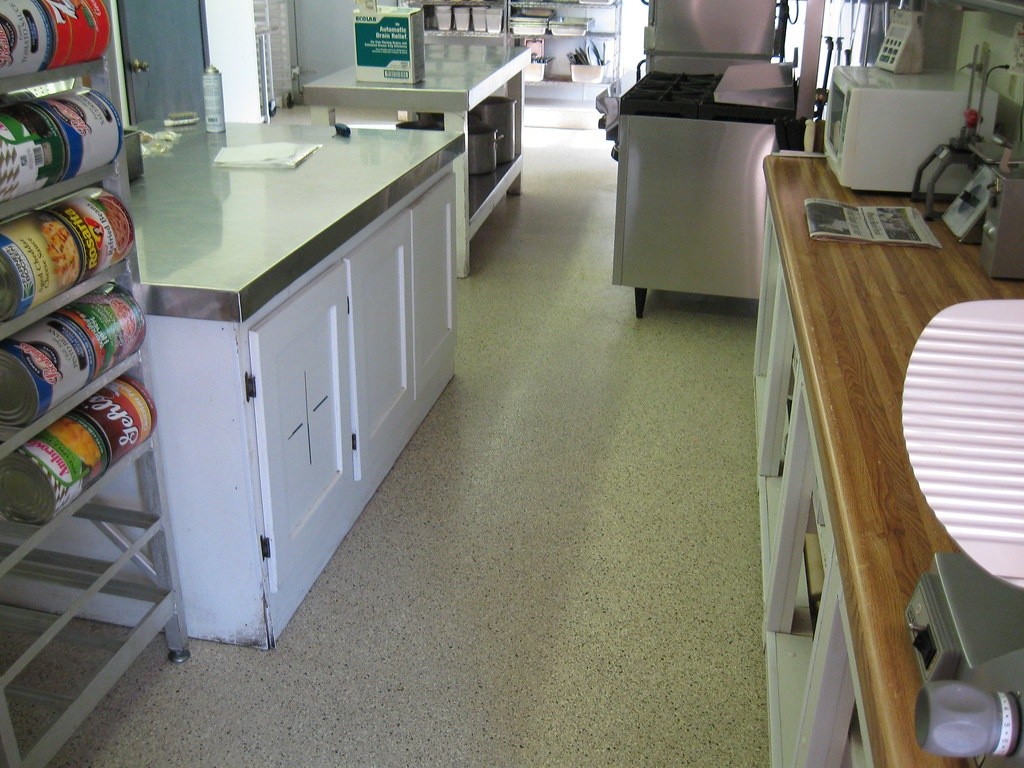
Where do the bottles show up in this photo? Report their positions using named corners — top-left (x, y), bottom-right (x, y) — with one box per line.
top-left (202, 64), bottom-right (226, 132)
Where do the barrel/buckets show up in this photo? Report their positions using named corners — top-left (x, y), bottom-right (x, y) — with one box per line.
top-left (470, 96), bottom-right (517, 162)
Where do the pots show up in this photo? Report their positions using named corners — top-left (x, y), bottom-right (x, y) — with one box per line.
top-left (468, 126), bottom-right (505, 174)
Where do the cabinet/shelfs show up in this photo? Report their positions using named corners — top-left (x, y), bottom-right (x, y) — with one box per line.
top-left (0, 117), bottom-right (465, 651)
top-left (508, 0), bottom-right (622, 130)
top-left (397, 0), bottom-right (512, 49)
top-left (0, 0), bottom-right (191, 768)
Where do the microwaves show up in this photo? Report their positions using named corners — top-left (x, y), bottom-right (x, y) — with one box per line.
top-left (824, 66), bottom-right (1000, 194)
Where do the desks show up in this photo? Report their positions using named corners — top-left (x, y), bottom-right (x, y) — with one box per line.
top-left (752, 155), bottom-right (1024, 768)
top-left (302, 44), bottom-right (532, 279)
